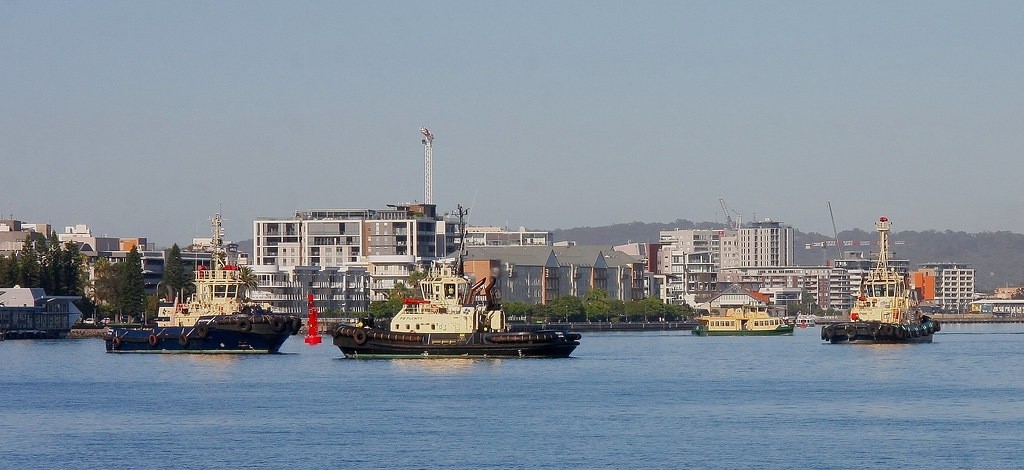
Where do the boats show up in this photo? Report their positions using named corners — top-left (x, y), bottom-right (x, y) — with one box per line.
top-left (104, 213), bottom-right (302, 354)
top-left (796, 313), bottom-right (815, 328)
top-left (691, 307), bottom-right (795, 336)
top-left (330, 203), bottom-right (581, 359)
top-left (821, 217), bottom-right (940, 344)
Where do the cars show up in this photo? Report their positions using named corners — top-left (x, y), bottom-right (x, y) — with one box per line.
top-left (85, 318), bottom-right (94, 324)
top-left (101, 318), bottom-right (111, 324)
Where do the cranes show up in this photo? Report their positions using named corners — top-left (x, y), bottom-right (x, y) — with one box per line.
top-left (420, 125), bottom-right (434, 204)
top-left (804, 240), bottom-right (910, 258)
top-left (719, 198), bottom-right (742, 230)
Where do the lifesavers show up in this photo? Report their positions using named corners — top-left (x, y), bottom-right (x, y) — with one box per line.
top-left (237, 319), bottom-right (252, 333)
top-left (271, 317), bottom-right (283, 331)
top-left (354, 329), bottom-right (366, 345)
top-left (292, 318), bottom-right (301, 331)
top-left (113, 337), bottom-right (120, 345)
top-left (195, 323), bottom-right (208, 338)
top-left (179, 334), bottom-right (188, 346)
top-left (820, 320), bottom-right (941, 342)
top-left (149, 334), bottom-right (157, 346)
